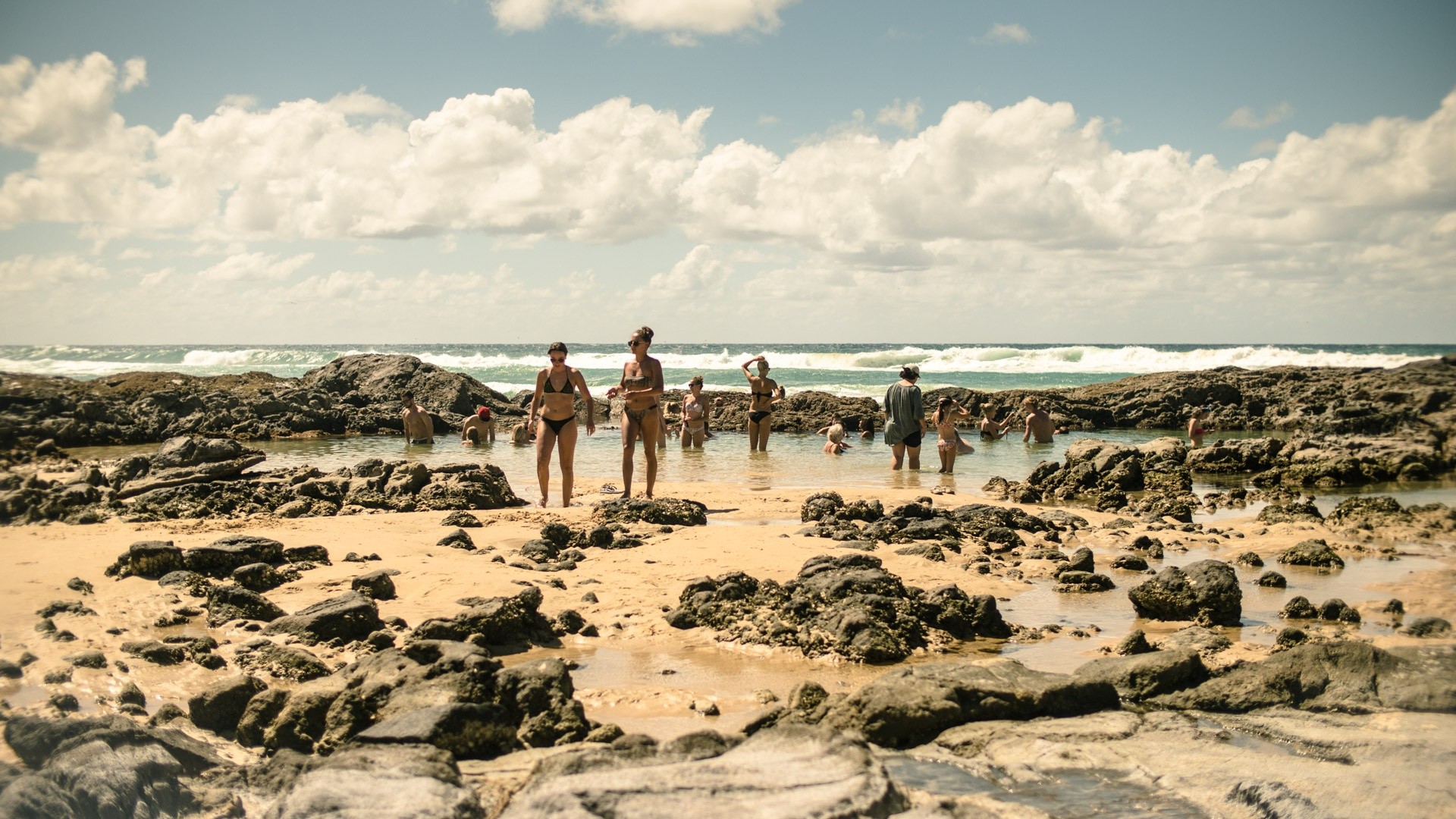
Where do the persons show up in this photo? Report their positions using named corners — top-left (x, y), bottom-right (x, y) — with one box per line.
top-left (1188, 404), bottom-right (1212, 448)
top-left (509, 423), bottom-right (529, 445)
top-left (823, 424), bottom-right (854, 455)
top-left (522, 421), bottom-right (538, 443)
top-left (604, 324), bottom-right (664, 499)
top-left (462, 406), bottom-right (495, 443)
top-left (858, 415), bottom-right (876, 441)
top-left (527, 341), bottom-right (596, 509)
top-left (881, 363), bottom-right (926, 470)
top-left (932, 392), bottom-right (970, 474)
top-left (663, 402), bottom-right (682, 439)
top-left (980, 402), bottom-right (1015, 443)
top-left (678, 375), bottom-right (710, 449)
top-left (1021, 394), bottom-right (1055, 443)
top-left (777, 385), bottom-right (786, 400)
top-left (400, 388), bottom-right (435, 446)
top-left (703, 421), bottom-right (715, 438)
top-left (953, 427), bottom-right (975, 455)
top-left (1052, 426), bottom-right (1070, 435)
top-left (818, 412), bottom-right (848, 439)
top-left (740, 355), bottom-right (782, 453)
top-left (461, 425), bottom-right (482, 446)
top-left (636, 395), bottom-right (667, 451)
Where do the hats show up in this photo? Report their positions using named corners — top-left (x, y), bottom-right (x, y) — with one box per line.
top-left (905, 365), bottom-right (921, 378)
top-left (478, 407), bottom-right (491, 422)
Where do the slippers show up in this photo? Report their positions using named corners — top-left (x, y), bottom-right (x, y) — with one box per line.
top-left (930, 485), bottom-right (942, 494)
top-left (600, 485), bottom-right (626, 493)
top-left (942, 485), bottom-right (955, 493)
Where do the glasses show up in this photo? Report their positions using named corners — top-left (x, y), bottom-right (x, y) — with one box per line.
top-left (550, 356), bottom-right (565, 362)
top-left (402, 397), bottom-right (413, 404)
top-left (689, 383), bottom-right (699, 386)
top-left (628, 341), bottom-right (647, 346)
top-left (915, 375), bottom-right (920, 380)
top-left (766, 368), bottom-right (771, 370)
